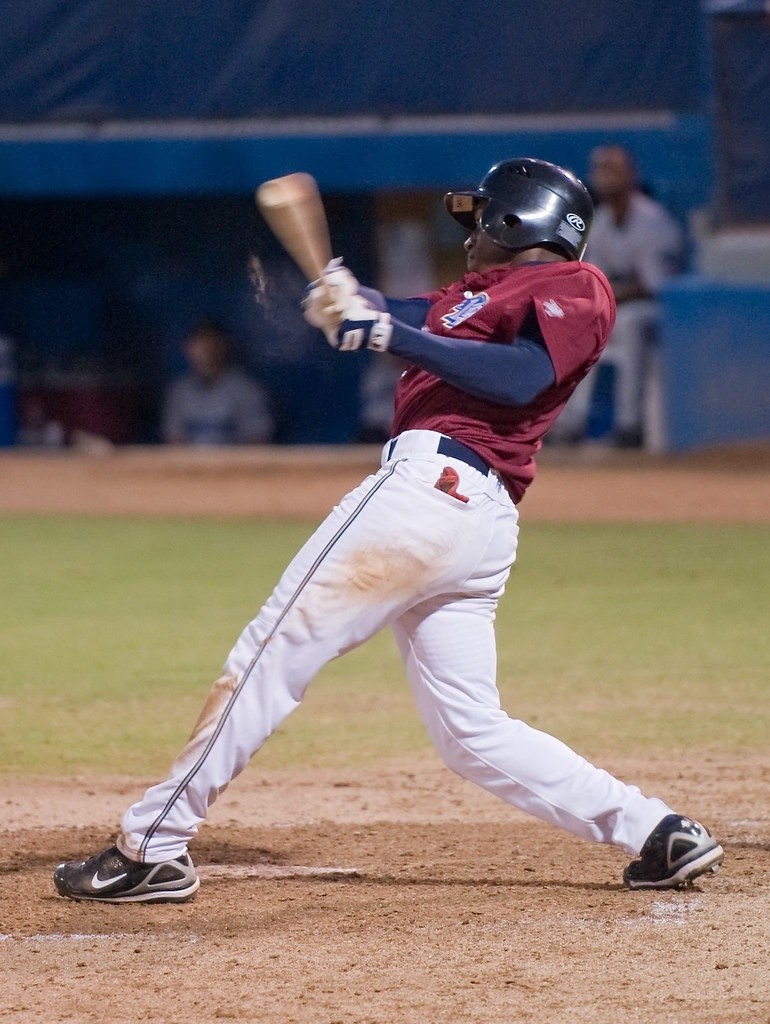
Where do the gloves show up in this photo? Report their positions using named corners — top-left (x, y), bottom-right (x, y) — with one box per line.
top-left (300, 258), bottom-right (394, 352)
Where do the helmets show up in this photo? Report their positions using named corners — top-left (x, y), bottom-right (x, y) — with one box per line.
top-left (444, 157), bottom-right (594, 263)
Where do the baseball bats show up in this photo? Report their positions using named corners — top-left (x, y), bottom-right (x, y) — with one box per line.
top-left (257, 167), bottom-right (350, 331)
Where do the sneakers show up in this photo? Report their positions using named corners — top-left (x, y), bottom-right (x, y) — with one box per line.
top-left (621, 813), bottom-right (725, 887)
top-left (53, 842), bottom-right (201, 903)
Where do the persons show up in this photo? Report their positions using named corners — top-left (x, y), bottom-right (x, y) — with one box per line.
top-left (53, 156), bottom-right (724, 904)
top-left (541, 142), bottom-right (685, 452)
top-left (0, 202), bottom-right (443, 445)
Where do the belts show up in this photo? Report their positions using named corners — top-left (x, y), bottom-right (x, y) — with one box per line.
top-left (388, 433), bottom-right (491, 477)
